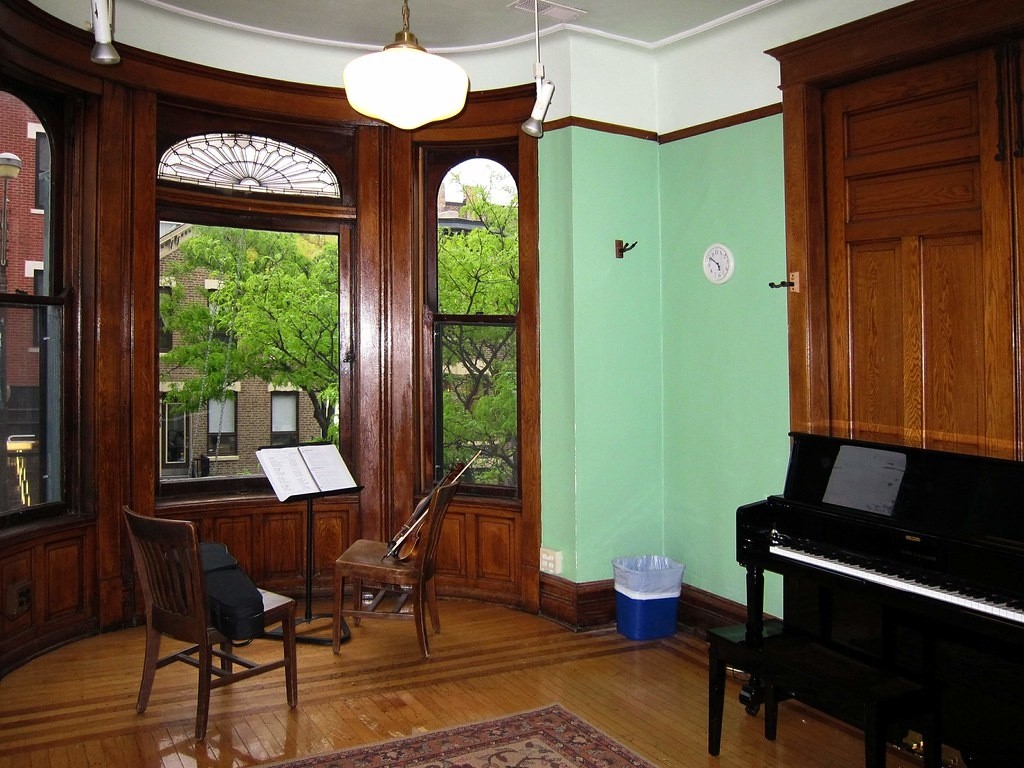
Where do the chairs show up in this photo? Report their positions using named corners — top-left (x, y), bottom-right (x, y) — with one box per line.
top-left (333, 480), bottom-right (462, 660)
top-left (122, 506), bottom-right (298, 741)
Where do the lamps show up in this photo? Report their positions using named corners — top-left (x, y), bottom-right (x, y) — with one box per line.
top-left (521, 0), bottom-right (555, 138)
top-left (345, 0), bottom-right (469, 131)
top-left (91, 0), bottom-right (121, 64)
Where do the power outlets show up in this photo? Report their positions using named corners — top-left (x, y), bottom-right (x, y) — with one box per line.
top-left (6, 580), bottom-right (33, 615)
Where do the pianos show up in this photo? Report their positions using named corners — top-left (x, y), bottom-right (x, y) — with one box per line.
top-left (734, 430), bottom-right (1024, 768)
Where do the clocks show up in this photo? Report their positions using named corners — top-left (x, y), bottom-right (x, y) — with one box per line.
top-left (702, 243), bottom-right (734, 285)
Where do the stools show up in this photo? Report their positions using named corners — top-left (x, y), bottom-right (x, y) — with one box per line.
top-left (705, 618), bottom-right (952, 768)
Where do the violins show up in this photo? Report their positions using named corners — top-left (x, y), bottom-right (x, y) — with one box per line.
top-left (386, 463), bottom-right (467, 563)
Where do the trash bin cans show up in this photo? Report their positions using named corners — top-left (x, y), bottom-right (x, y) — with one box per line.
top-left (612, 556), bottom-right (686, 641)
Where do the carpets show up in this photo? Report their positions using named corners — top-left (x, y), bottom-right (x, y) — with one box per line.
top-left (249, 702), bottom-right (662, 768)
